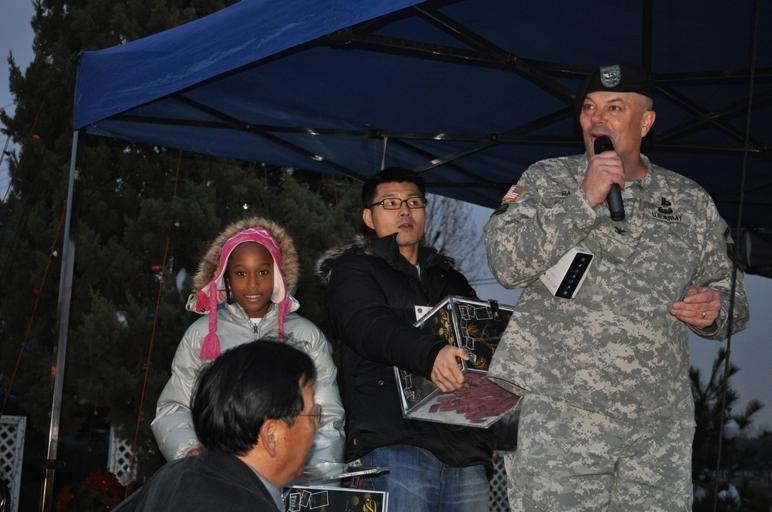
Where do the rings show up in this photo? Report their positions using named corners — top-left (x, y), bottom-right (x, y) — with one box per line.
top-left (702, 311), bottom-right (707, 321)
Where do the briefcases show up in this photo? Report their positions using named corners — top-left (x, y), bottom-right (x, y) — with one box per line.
top-left (391, 294), bottom-right (531, 428)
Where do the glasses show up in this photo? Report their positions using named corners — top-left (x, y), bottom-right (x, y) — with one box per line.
top-left (294, 402), bottom-right (324, 425)
top-left (370, 195), bottom-right (429, 212)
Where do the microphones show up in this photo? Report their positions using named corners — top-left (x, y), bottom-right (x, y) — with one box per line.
top-left (593, 134), bottom-right (626, 221)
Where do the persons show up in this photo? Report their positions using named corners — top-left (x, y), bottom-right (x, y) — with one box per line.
top-left (483, 49), bottom-right (750, 511)
top-left (314, 166), bottom-right (494, 512)
top-left (150, 217), bottom-right (346, 488)
top-left (116, 339), bottom-right (322, 510)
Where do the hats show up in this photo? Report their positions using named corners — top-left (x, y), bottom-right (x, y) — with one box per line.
top-left (202, 226), bottom-right (291, 359)
top-left (584, 61), bottom-right (658, 102)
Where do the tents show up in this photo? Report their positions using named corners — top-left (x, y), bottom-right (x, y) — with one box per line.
top-left (38, 4), bottom-right (770, 512)
